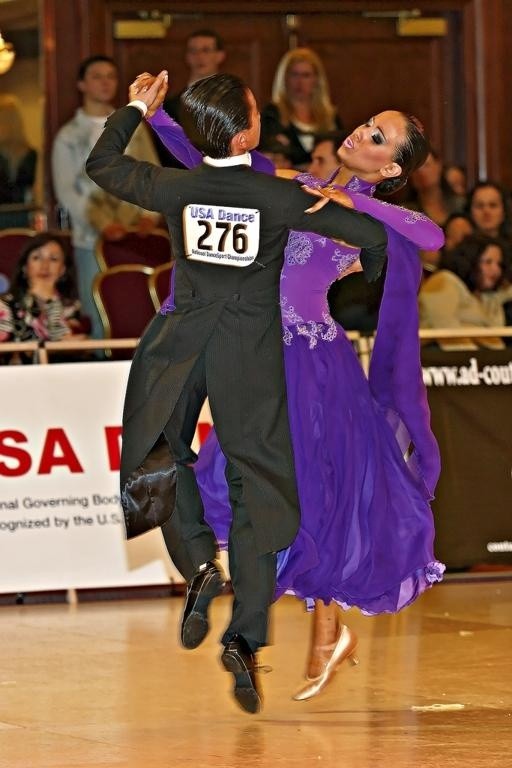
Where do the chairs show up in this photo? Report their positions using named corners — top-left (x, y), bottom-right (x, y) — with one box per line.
top-left (149, 257), bottom-right (174, 312)
top-left (0, 228), bottom-right (42, 286)
top-left (94, 223), bottom-right (173, 272)
top-left (91, 264), bottom-right (153, 356)
top-left (34, 234), bottom-right (76, 300)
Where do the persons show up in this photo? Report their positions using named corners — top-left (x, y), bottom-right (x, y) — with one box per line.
top-left (153, 27), bottom-right (226, 168)
top-left (406, 150), bottom-right (512, 351)
top-left (258, 47), bottom-right (342, 172)
top-left (86, 68), bottom-right (388, 715)
top-left (307, 131), bottom-right (344, 184)
top-left (0, 232), bottom-right (93, 365)
top-left (130, 72), bottom-right (446, 703)
top-left (1, 94), bottom-right (40, 232)
top-left (53, 56), bottom-right (165, 359)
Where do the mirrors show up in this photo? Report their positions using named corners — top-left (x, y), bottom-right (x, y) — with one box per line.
top-left (0, 2), bottom-right (50, 218)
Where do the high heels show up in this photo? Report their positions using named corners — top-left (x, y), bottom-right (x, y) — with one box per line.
top-left (294, 623), bottom-right (358, 702)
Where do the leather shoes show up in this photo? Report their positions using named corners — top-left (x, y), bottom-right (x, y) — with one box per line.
top-left (182, 563), bottom-right (228, 650)
top-left (222, 634), bottom-right (265, 714)
